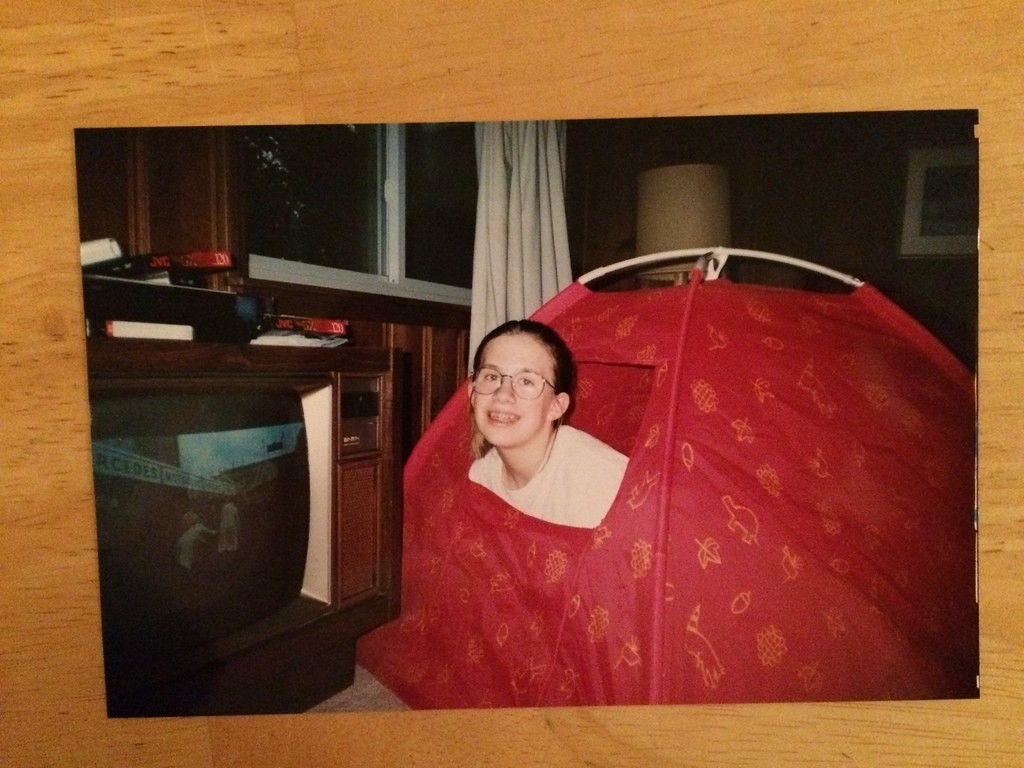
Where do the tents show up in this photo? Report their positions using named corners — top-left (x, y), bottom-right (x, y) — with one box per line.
top-left (354, 248), bottom-right (974, 709)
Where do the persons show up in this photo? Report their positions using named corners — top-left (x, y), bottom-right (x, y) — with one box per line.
top-left (469, 320), bottom-right (629, 529)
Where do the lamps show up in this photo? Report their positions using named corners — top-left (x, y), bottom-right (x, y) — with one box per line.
top-left (635, 163), bottom-right (732, 288)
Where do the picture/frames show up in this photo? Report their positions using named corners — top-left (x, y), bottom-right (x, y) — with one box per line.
top-left (894, 140), bottom-right (979, 262)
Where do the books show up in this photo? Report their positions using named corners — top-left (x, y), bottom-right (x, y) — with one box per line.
top-left (105, 320), bottom-right (193, 341)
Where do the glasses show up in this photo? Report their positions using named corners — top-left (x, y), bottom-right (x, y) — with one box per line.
top-left (472, 367), bottom-right (557, 401)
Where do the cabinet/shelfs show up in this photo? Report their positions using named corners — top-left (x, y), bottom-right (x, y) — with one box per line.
top-left (334, 318), bottom-right (470, 612)
